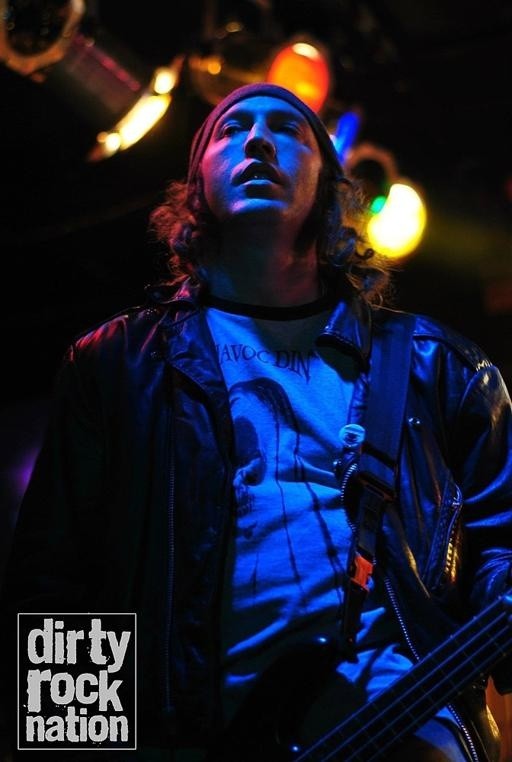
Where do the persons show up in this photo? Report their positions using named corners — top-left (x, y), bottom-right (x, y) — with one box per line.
top-left (0, 84), bottom-right (511, 762)
